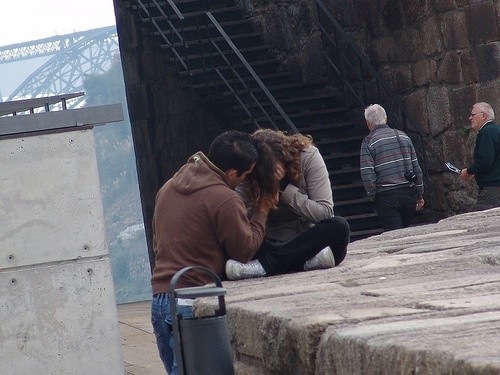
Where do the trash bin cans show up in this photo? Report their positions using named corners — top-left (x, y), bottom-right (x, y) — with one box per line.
top-left (168, 264), bottom-right (234, 375)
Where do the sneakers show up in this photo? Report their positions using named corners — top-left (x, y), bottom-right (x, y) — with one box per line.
top-left (303, 245), bottom-right (335, 271)
top-left (225, 258), bottom-right (266, 280)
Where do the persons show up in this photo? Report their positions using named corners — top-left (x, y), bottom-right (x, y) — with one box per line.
top-left (151, 133), bottom-right (278, 375)
top-left (224, 128), bottom-right (350, 278)
top-left (360, 104), bottom-right (424, 231)
top-left (458, 102), bottom-right (500, 210)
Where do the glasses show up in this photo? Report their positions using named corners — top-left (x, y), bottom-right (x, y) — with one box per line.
top-left (468, 111), bottom-right (484, 117)
